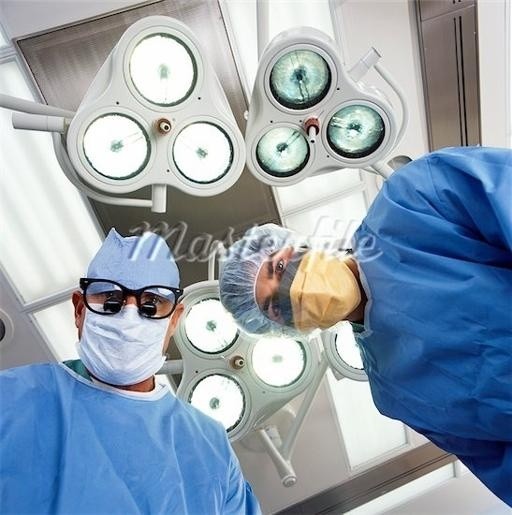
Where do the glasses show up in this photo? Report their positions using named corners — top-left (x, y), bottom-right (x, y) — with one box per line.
top-left (77, 276), bottom-right (186, 319)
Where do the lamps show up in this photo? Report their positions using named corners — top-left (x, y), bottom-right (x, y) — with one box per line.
top-left (246, 24), bottom-right (409, 188)
top-left (1, 15), bottom-right (246, 214)
top-left (156, 239), bottom-right (370, 489)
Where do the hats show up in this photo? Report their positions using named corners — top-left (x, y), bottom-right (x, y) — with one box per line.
top-left (87, 228), bottom-right (180, 303)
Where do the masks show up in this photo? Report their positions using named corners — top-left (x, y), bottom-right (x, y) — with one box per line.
top-left (73, 303), bottom-right (178, 387)
top-left (275, 247), bottom-right (363, 335)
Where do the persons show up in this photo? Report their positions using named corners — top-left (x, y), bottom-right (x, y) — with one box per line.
top-left (218, 146), bottom-right (512, 507)
top-left (0, 227), bottom-right (264, 515)
